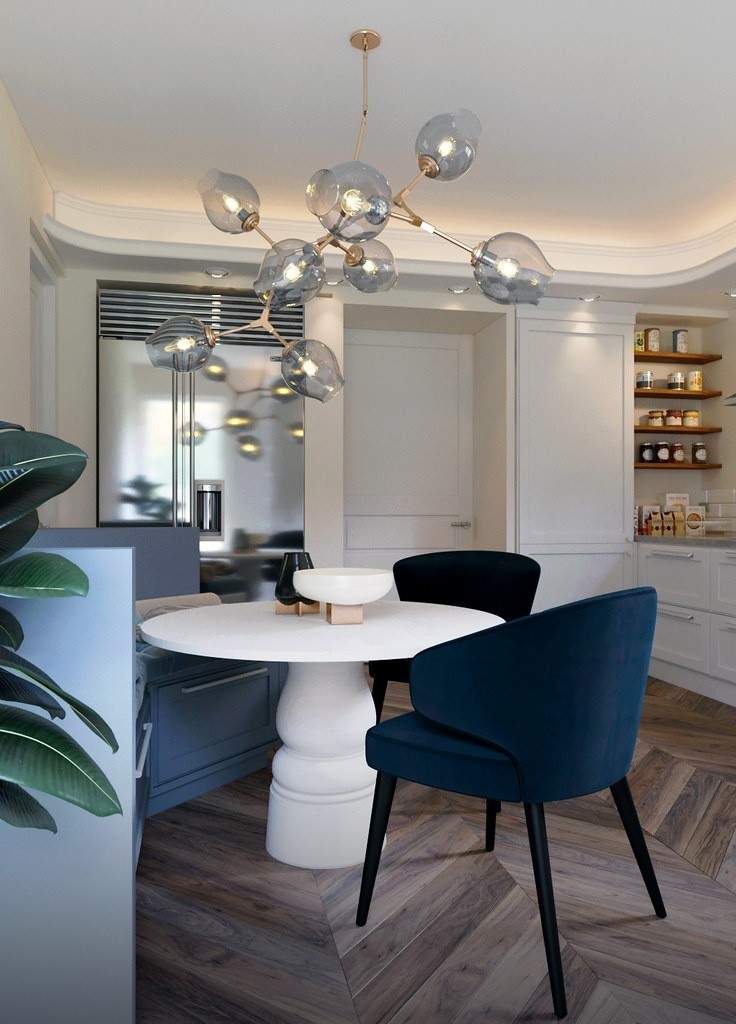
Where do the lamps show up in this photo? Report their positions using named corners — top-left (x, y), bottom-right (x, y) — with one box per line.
top-left (177, 355), bottom-right (304, 460)
top-left (145, 30), bottom-right (555, 403)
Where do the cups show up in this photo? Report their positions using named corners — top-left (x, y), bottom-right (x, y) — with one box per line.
top-left (636, 372), bottom-right (653, 389)
top-left (634, 328), bottom-right (644, 353)
top-left (685, 371), bottom-right (702, 392)
top-left (665, 371), bottom-right (683, 391)
top-left (644, 328), bottom-right (659, 353)
top-left (671, 330), bottom-right (688, 353)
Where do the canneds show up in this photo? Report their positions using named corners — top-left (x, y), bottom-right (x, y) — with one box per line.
top-left (634, 327), bottom-right (708, 465)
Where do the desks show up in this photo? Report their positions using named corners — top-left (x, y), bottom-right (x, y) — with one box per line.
top-left (200, 548), bottom-right (303, 600)
top-left (140, 601), bottom-right (507, 870)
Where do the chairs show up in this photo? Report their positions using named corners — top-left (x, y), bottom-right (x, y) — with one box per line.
top-left (355, 587), bottom-right (668, 1018)
top-left (368, 550), bottom-right (541, 725)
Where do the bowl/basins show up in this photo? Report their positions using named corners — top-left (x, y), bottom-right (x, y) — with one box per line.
top-left (292, 567), bottom-right (394, 605)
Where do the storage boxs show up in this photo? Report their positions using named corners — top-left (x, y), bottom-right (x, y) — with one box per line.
top-left (634, 493), bottom-right (705, 536)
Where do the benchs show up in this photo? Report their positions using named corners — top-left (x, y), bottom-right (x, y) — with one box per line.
top-left (20, 527), bottom-right (288, 817)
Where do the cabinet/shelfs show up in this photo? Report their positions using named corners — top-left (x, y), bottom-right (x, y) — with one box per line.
top-left (515, 319), bottom-right (634, 616)
top-left (633, 351), bottom-right (723, 470)
top-left (637, 542), bottom-right (736, 707)
